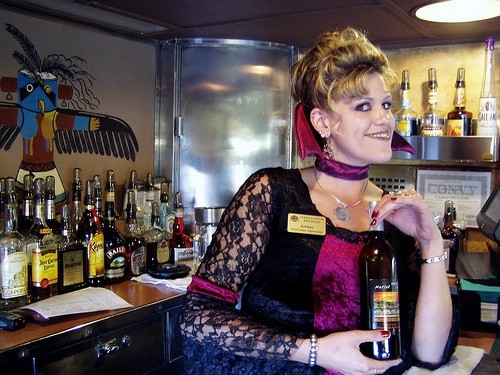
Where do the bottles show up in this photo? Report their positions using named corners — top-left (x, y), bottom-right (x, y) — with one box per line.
top-left (393, 70), bottom-right (419, 136)
top-left (445, 67), bottom-right (472, 136)
top-left (359, 200), bottom-right (400, 360)
top-left (0, 168), bottom-right (195, 319)
top-left (476, 36), bottom-right (499, 163)
top-left (440, 200), bottom-right (464, 274)
top-left (420, 67), bottom-right (445, 137)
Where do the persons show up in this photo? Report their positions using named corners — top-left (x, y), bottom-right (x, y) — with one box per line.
top-left (178, 27), bottom-right (460, 375)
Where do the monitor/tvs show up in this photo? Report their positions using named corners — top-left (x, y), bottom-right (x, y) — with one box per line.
top-left (476, 182), bottom-right (500, 240)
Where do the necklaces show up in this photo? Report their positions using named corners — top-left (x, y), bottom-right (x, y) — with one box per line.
top-left (313, 166), bottom-right (368, 223)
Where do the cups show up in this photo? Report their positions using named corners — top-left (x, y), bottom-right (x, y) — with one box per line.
top-left (194, 207), bottom-right (227, 271)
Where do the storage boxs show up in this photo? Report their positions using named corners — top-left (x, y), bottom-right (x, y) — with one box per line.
top-left (437, 256), bottom-right (500, 333)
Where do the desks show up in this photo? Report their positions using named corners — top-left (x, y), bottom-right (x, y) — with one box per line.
top-left (1, 258), bottom-right (201, 375)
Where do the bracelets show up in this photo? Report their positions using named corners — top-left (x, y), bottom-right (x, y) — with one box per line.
top-left (420, 251), bottom-right (448, 264)
top-left (308, 334), bottom-right (317, 367)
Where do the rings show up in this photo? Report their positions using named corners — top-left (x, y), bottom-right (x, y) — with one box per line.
top-left (373, 370), bottom-right (376, 375)
top-left (389, 187), bottom-right (410, 197)
